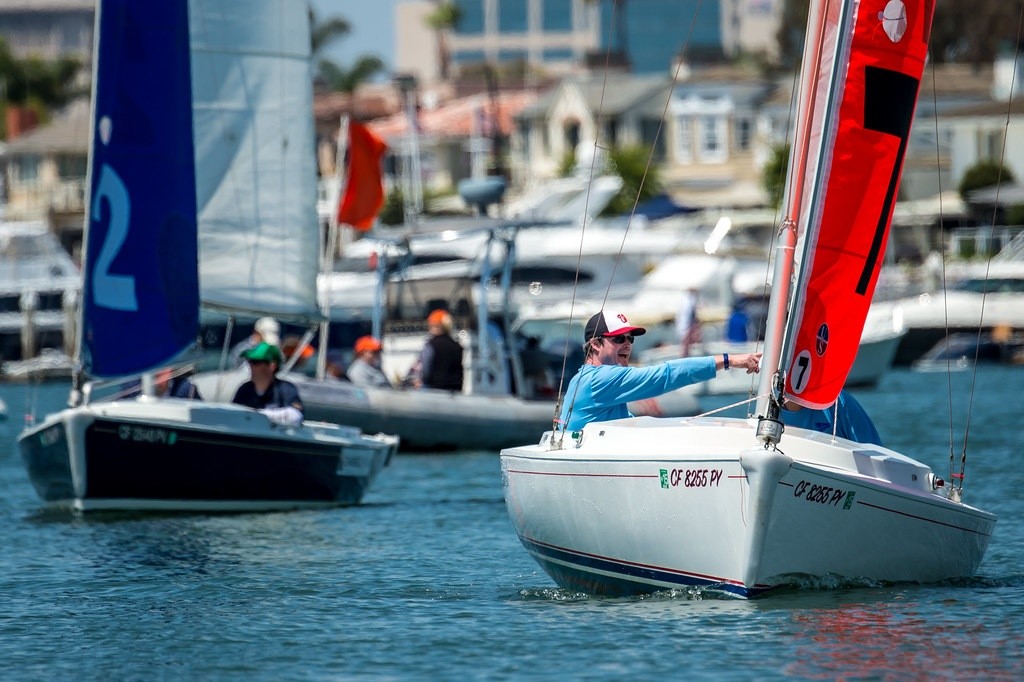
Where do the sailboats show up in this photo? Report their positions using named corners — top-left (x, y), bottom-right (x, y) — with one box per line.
top-left (499, 0), bottom-right (998, 603)
top-left (17, 0), bottom-right (400, 518)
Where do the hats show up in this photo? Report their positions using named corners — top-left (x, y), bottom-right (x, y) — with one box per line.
top-left (284, 337), bottom-right (315, 358)
top-left (355, 335), bottom-right (383, 355)
top-left (426, 310), bottom-right (452, 324)
top-left (240, 342), bottom-right (282, 371)
top-left (585, 310), bottom-right (646, 342)
top-left (254, 316), bottom-right (280, 346)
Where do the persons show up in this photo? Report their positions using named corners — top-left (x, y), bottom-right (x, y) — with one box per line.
top-left (778, 387), bottom-right (881, 445)
top-left (346, 336), bottom-right (391, 388)
top-left (410, 309), bottom-right (463, 392)
top-left (673, 288), bottom-right (700, 358)
top-left (558, 310), bottom-right (761, 432)
top-left (225, 317), bottom-right (317, 377)
top-left (724, 296), bottom-right (755, 343)
top-left (119, 368), bottom-right (201, 400)
top-left (232, 342), bottom-right (306, 417)
top-left (509, 336), bottom-right (539, 394)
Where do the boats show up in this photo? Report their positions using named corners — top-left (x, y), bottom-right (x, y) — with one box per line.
top-left (0, 174), bottom-right (1023, 454)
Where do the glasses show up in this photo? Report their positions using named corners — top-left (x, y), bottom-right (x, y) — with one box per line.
top-left (596, 334), bottom-right (634, 344)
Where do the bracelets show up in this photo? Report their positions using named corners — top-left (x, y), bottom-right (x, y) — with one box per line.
top-left (723, 353), bottom-right (730, 370)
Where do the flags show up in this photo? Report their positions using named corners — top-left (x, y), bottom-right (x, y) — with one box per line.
top-left (339, 119), bottom-right (387, 231)
